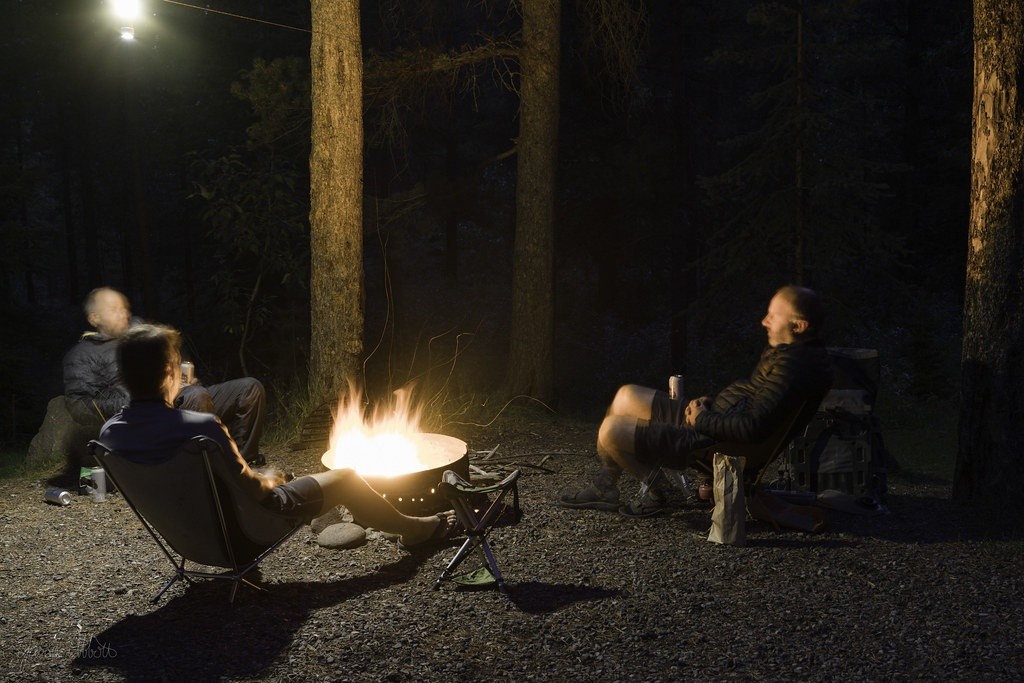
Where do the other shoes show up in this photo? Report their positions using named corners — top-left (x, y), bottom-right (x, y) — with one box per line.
top-left (248, 454), bottom-right (265, 468)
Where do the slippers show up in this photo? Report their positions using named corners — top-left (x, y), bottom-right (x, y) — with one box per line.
top-left (618, 485), bottom-right (677, 519)
top-left (560, 480), bottom-right (625, 512)
top-left (397, 509), bottom-right (462, 555)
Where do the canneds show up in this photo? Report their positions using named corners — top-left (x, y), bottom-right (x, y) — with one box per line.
top-left (91, 467), bottom-right (106, 504)
top-left (181, 361), bottom-right (195, 385)
top-left (669, 375), bottom-right (684, 400)
top-left (44, 488), bottom-right (71, 506)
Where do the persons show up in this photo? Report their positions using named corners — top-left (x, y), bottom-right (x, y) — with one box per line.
top-left (63, 287), bottom-right (269, 467)
top-left (99, 322), bottom-right (462, 565)
top-left (560, 286), bottom-right (820, 518)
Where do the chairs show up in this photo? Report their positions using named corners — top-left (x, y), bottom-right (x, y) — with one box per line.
top-left (87, 434), bottom-right (305, 612)
top-left (648, 373), bottom-right (829, 530)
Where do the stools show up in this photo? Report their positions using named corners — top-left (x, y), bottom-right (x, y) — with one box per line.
top-left (433, 469), bottom-right (520, 593)
